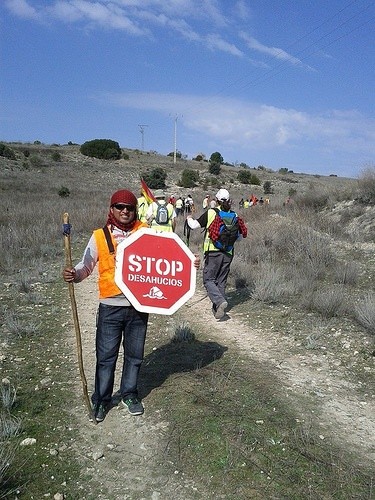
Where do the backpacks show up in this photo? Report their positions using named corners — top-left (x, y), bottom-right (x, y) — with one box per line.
top-left (211, 208), bottom-right (239, 252)
top-left (155, 201), bottom-right (172, 225)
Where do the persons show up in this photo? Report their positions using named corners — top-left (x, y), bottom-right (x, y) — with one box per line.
top-left (63, 190), bottom-right (201, 422)
top-left (238, 197), bottom-right (274, 211)
top-left (187, 189), bottom-right (239, 319)
top-left (203, 194), bottom-right (210, 211)
top-left (210, 198), bottom-right (216, 208)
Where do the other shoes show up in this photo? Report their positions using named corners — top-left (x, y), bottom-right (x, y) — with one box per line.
top-left (214, 302), bottom-right (228, 318)
top-left (212, 304), bottom-right (218, 316)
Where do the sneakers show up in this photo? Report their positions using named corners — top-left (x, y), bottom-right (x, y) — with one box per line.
top-left (122, 398), bottom-right (144, 415)
top-left (89, 404), bottom-right (105, 422)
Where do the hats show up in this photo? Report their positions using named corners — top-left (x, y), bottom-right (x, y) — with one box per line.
top-left (153, 189), bottom-right (166, 198)
top-left (171, 195), bottom-right (174, 198)
top-left (206, 195), bottom-right (210, 197)
top-left (215, 188), bottom-right (230, 202)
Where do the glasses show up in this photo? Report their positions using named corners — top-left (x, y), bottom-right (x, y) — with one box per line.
top-left (111, 204), bottom-right (134, 212)
